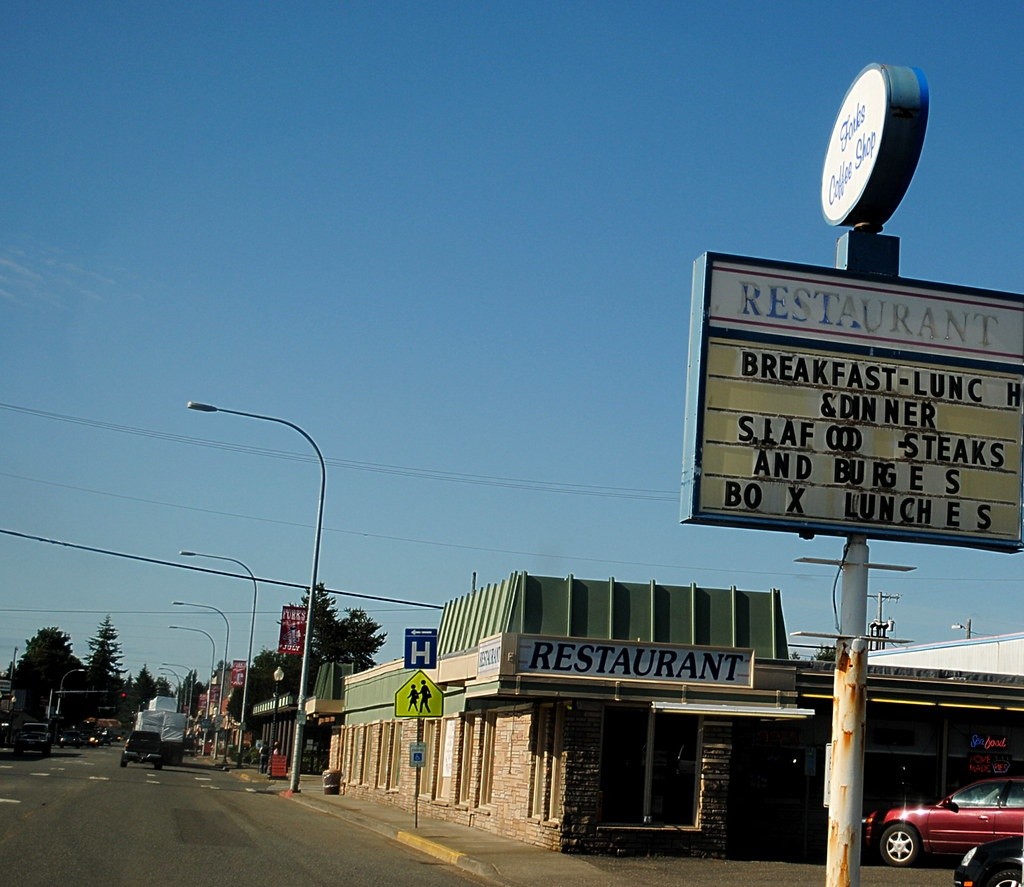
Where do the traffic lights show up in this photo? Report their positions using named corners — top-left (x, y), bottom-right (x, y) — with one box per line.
top-left (122, 693), bottom-right (126, 697)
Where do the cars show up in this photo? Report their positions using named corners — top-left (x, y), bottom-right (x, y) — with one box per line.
top-left (184, 735), bottom-right (197, 756)
top-left (14, 723), bottom-right (53, 756)
top-left (953, 836), bottom-right (1024, 887)
top-left (60, 731), bottom-right (82, 749)
top-left (861, 777), bottom-right (1024, 868)
top-left (78, 727), bottom-right (112, 747)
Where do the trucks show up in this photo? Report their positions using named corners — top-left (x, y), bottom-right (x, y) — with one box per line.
top-left (133, 696), bottom-right (187, 766)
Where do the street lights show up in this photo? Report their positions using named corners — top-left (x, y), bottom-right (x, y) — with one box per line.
top-left (180, 551), bottom-right (257, 768)
top-left (8, 696), bottom-right (16, 748)
top-left (173, 601), bottom-right (229, 760)
top-left (187, 401), bottom-right (325, 794)
top-left (53, 669), bottom-right (85, 745)
top-left (157, 662), bottom-right (194, 728)
top-left (169, 625), bottom-right (215, 756)
top-left (267, 667), bottom-right (284, 774)
top-left (10, 647), bottom-right (18, 679)
top-left (951, 619), bottom-right (971, 639)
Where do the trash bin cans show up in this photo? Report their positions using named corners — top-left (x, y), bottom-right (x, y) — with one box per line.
top-left (322, 769), bottom-right (342, 795)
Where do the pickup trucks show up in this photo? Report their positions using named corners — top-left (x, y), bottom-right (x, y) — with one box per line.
top-left (120, 731), bottom-right (164, 770)
